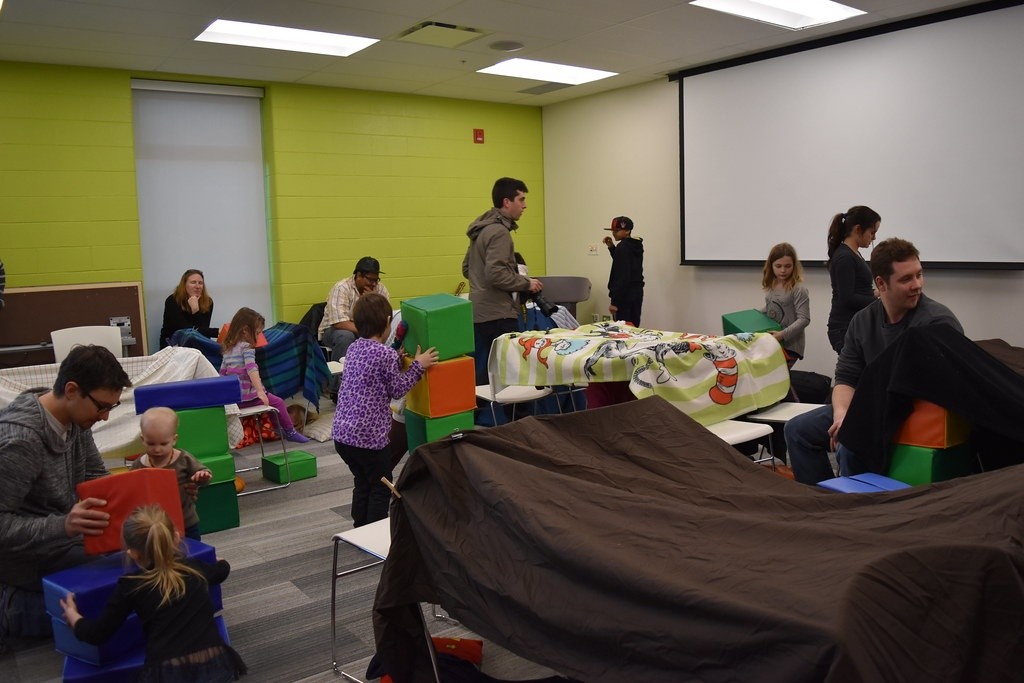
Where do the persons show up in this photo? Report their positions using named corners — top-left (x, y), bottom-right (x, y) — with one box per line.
top-left (158, 269), bottom-right (214, 352)
top-left (785, 237), bottom-right (964, 486)
top-left (130, 406), bottom-right (211, 542)
top-left (220, 307), bottom-right (309, 442)
top-left (0, 344), bottom-right (132, 636)
top-left (332, 292), bottom-right (439, 528)
top-left (462, 177), bottom-right (543, 416)
top-left (60, 506), bottom-right (248, 683)
top-left (318, 256), bottom-right (389, 401)
top-left (759, 242), bottom-right (810, 373)
top-left (827, 205), bottom-right (881, 355)
top-left (603, 217), bottom-right (645, 330)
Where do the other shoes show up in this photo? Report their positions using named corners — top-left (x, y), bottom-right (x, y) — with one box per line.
top-left (330, 394), bottom-right (337, 405)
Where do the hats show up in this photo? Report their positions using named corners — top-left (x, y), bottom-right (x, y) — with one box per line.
top-left (353, 257), bottom-right (385, 274)
top-left (604, 216), bottom-right (634, 230)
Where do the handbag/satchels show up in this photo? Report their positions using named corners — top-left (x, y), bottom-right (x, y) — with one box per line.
top-left (780, 370), bottom-right (831, 403)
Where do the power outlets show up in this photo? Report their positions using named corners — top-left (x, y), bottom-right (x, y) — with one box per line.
top-left (593, 314), bottom-right (599, 322)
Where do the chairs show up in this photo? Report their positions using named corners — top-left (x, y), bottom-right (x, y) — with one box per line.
top-left (50, 326), bottom-right (123, 364)
top-left (551, 382), bottom-right (590, 416)
top-left (475, 384), bottom-right (553, 428)
top-left (329, 322), bottom-right (1024, 683)
top-left (235, 405), bottom-right (291, 496)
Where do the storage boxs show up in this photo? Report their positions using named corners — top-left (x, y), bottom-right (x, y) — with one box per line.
top-left (403, 409), bottom-right (475, 443)
top-left (134, 374), bottom-right (243, 416)
top-left (896, 401), bottom-right (976, 448)
top-left (402, 355), bottom-right (478, 419)
top-left (399, 293), bottom-right (476, 360)
top-left (721, 308), bottom-right (784, 349)
top-left (61, 613), bottom-right (232, 683)
top-left (41, 535), bottom-right (219, 628)
top-left (196, 454), bottom-right (235, 486)
top-left (849, 473), bottom-right (914, 492)
top-left (407, 433), bottom-right (423, 455)
top-left (261, 449), bottom-right (317, 484)
top-left (195, 481), bottom-right (240, 536)
top-left (78, 467), bottom-right (185, 558)
top-left (887, 444), bottom-right (970, 487)
top-left (107, 466), bottom-right (130, 474)
top-left (815, 476), bottom-right (887, 494)
top-left (173, 406), bottom-right (229, 458)
top-left (50, 584), bottom-right (223, 668)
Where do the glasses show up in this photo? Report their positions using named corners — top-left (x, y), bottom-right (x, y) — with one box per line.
top-left (363, 274), bottom-right (380, 283)
top-left (88, 394), bottom-right (120, 413)
